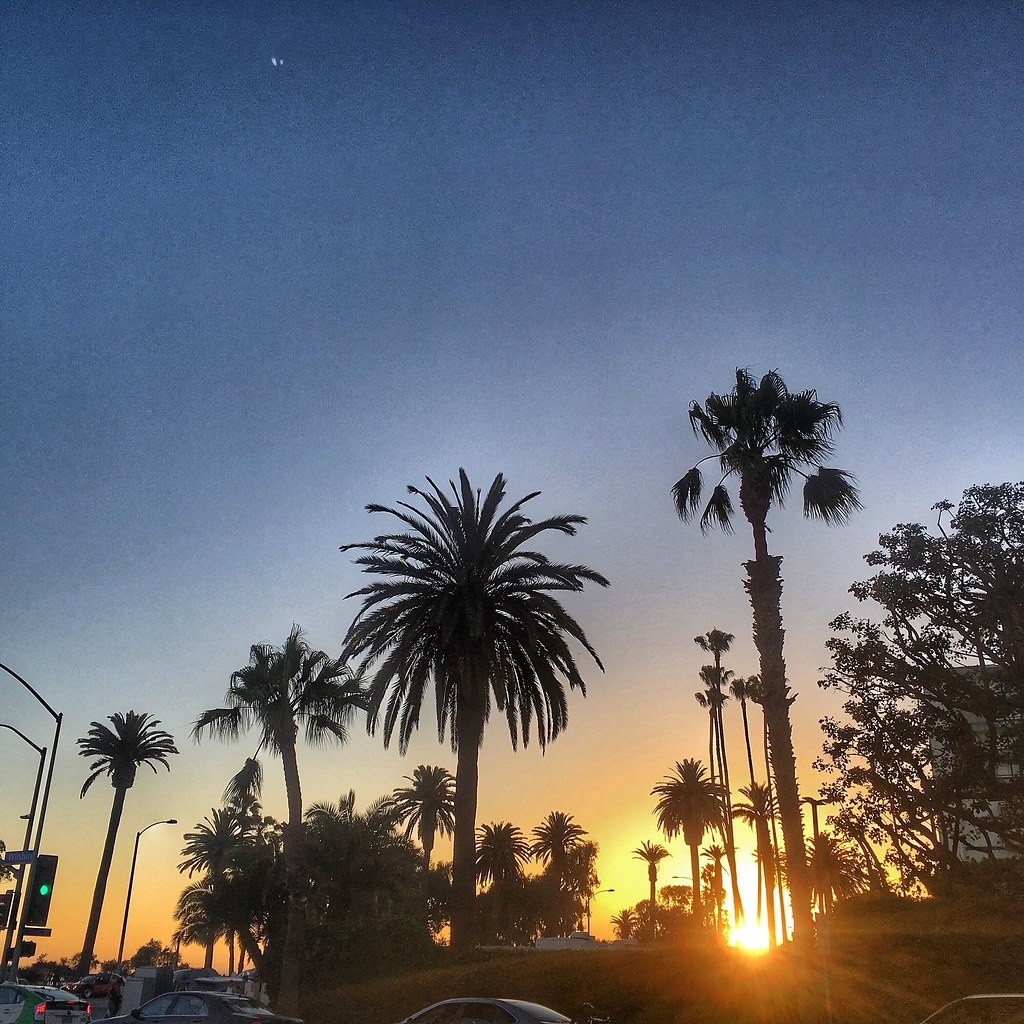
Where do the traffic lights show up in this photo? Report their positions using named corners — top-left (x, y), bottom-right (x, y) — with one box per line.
top-left (0, 893), bottom-right (11, 930)
top-left (25, 853), bottom-right (58, 926)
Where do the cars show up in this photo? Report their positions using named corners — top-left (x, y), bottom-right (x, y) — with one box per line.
top-left (182, 977), bottom-right (251, 997)
top-left (237, 970), bottom-right (257, 979)
top-left (916, 995), bottom-right (1024, 1024)
top-left (84, 991), bottom-right (302, 1024)
top-left (66, 973), bottom-right (125, 997)
top-left (0, 984), bottom-right (91, 1024)
top-left (389, 999), bottom-right (573, 1024)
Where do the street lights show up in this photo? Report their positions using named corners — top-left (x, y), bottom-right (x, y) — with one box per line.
top-left (586, 888), bottom-right (614, 942)
top-left (669, 875), bottom-right (711, 944)
top-left (109, 819), bottom-right (180, 976)
